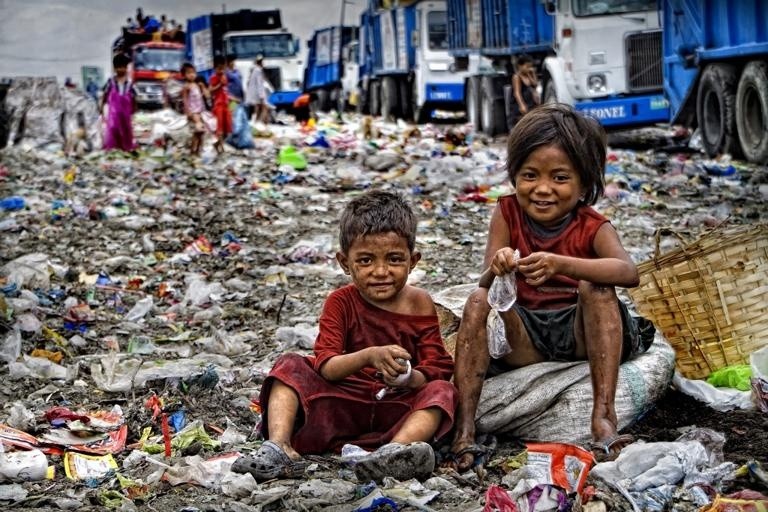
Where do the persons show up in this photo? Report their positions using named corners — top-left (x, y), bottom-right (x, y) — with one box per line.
top-left (119, 6), bottom-right (184, 43)
top-left (180, 63), bottom-right (208, 160)
top-left (438, 100), bottom-right (656, 475)
top-left (222, 56), bottom-right (257, 149)
top-left (228, 188), bottom-right (462, 486)
top-left (291, 92), bottom-right (319, 134)
top-left (208, 54), bottom-right (234, 154)
top-left (242, 53), bottom-right (275, 132)
top-left (98, 52), bottom-right (141, 158)
top-left (509, 53), bottom-right (538, 130)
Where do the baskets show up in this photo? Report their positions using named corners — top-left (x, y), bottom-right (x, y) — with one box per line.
top-left (624, 214), bottom-right (768, 382)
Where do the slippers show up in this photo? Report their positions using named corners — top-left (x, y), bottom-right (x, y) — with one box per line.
top-left (588, 433), bottom-right (639, 463)
top-left (444, 433), bottom-right (501, 475)
top-left (231, 441), bottom-right (308, 482)
top-left (358, 441), bottom-right (440, 484)
